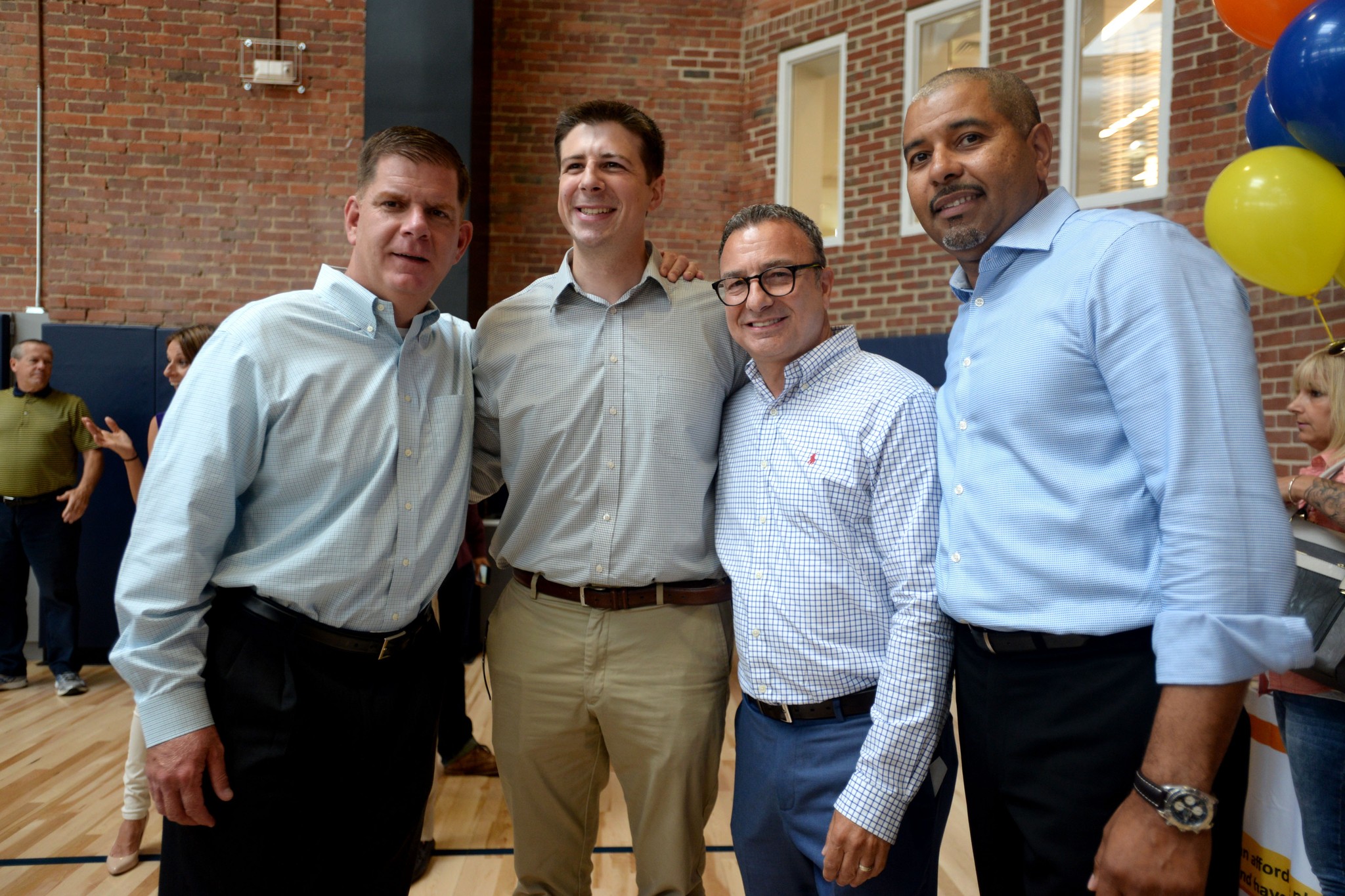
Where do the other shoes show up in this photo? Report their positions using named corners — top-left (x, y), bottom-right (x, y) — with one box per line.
top-left (105, 813), bottom-right (150, 874)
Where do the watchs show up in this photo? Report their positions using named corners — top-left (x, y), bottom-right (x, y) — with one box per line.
top-left (1133, 769), bottom-right (1221, 834)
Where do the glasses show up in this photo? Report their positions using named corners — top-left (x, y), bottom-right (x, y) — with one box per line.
top-left (712, 263), bottom-right (824, 306)
top-left (1326, 341), bottom-right (1345, 357)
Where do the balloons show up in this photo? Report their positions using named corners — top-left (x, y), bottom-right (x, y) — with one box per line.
top-left (1212, 0), bottom-right (1345, 178)
top-left (1204, 147), bottom-right (1345, 297)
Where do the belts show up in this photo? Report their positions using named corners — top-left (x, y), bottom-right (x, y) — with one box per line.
top-left (511, 567), bottom-right (732, 609)
top-left (958, 625), bottom-right (1107, 652)
top-left (743, 690), bottom-right (872, 723)
top-left (245, 597), bottom-right (430, 660)
top-left (0, 494), bottom-right (56, 506)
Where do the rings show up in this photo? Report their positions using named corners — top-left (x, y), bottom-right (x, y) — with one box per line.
top-left (859, 864), bottom-right (873, 872)
top-left (78, 511), bottom-right (80, 514)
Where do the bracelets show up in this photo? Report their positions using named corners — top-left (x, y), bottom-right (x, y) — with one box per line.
top-left (122, 454), bottom-right (138, 462)
top-left (1288, 474), bottom-right (1305, 506)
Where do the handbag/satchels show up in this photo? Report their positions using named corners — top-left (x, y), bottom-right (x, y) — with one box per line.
top-left (1283, 458), bottom-right (1345, 671)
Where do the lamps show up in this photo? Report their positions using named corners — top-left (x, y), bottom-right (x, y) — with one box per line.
top-left (253, 58), bottom-right (294, 86)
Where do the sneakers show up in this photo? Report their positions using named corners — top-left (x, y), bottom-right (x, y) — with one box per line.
top-left (0, 673), bottom-right (28, 690)
top-left (54, 672), bottom-right (88, 696)
top-left (443, 744), bottom-right (500, 776)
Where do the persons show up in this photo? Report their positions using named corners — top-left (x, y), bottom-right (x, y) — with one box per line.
top-left (472, 99), bottom-right (752, 896)
top-left (108, 140), bottom-right (706, 896)
top-left (1261, 341), bottom-right (1345, 896)
top-left (437, 507), bottom-right (499, 777)
top-left (712, 201), bottom-right (959, 896)
top-left (80, 323), bottom-right (221, 875)
top-left (0, 339), bottom-right (105, 696)
top-left (902, 67), bottom-right (1310, 896)
